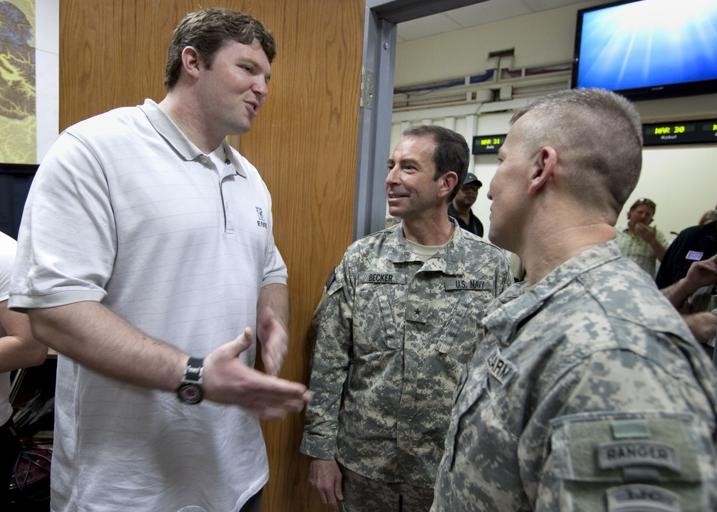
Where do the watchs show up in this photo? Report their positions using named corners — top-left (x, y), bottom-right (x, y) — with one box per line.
top-left (175, 356), bottom-right (208, 406)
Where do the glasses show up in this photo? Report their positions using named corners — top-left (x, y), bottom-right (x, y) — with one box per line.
top-left (632, 197), bottom-right (658, 210)
top-left (461, 183), bottom-right (480, 191)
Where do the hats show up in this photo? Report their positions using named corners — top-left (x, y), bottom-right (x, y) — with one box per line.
top-left (462, 173), bottom-right (483, 191)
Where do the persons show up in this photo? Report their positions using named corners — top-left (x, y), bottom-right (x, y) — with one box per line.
top-left (424, 85), bottom-right (717, 512)
top-left (300, 121), bottom-right (516, 511)
top-left (8, 3), bottom-right (317, 510)
top-left (613, 195), bottom-right (717, 362)
top-left (1, 228), bottom-right (48, 375)
top-left (445, 170), bottom-right (486, 240)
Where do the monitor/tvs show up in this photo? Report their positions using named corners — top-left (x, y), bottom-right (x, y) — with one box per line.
top-left (570, 0), bottom-right (717, 102)
